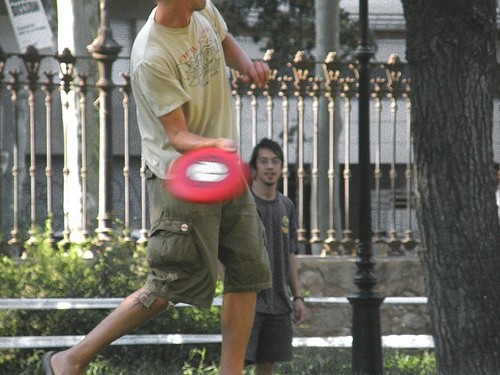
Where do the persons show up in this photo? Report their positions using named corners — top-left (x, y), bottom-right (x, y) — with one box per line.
top-left (42, 0), bottom-right (273, 375)
top-left (244, 138), bottom-right (306, 375)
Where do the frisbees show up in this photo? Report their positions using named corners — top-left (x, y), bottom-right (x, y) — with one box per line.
top-left (167, 148), bottom-right (252, 203)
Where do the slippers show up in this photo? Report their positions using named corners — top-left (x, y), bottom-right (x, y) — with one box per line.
top-left (42, 349), bottom-right (56, 375)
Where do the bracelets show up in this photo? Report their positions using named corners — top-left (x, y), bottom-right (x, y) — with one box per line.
top-left (294, 296), bottom-right (304, 303)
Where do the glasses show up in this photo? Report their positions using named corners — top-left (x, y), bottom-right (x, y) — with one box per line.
top-left (256, 159), bottom-right (280, 165)
